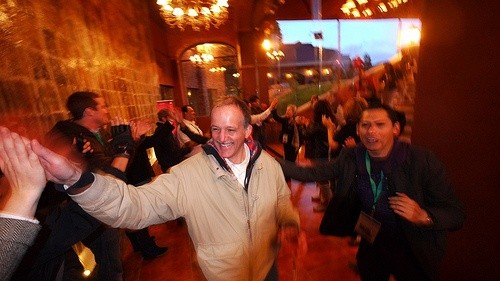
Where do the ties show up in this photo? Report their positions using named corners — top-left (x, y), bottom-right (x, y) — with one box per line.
top-left (191, 124), bottom-right (199, 133)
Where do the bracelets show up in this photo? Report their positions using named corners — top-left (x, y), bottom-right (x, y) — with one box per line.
top-left (425, 217), bottom-right (432, 225)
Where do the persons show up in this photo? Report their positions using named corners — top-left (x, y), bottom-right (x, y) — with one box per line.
top-left (20, 95), bottom-right (299, 281)
top-left (244, 44), bottom-right (417, 212)
top-left (267, 105), bottom-right (464, 281)
top-left (0, 91), bottom-right (211, 281)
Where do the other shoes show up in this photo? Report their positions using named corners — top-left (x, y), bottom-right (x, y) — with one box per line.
top-left (313, 207), bottom-right (324, 212)
top-left (134, 236), bottom-right (154, 251)
top-left (144, 247), bottom-right (166, 260)
top-left (312, 196), bottom-right (321, 201)
